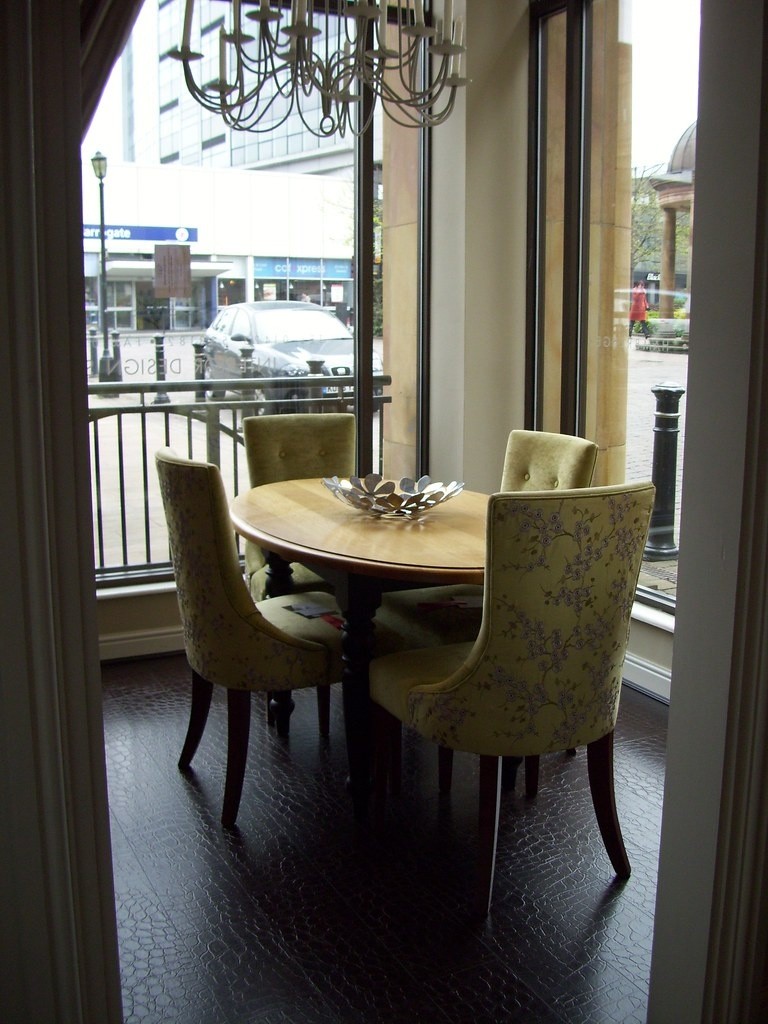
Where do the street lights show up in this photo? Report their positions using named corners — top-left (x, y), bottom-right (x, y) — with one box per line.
top-left (89, 151), bottom-right (120, 399)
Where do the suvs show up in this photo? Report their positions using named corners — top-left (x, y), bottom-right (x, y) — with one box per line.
top-left (201, 303), bottom-right (384, 419)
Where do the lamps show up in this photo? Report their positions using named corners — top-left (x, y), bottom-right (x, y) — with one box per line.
top-left (168, 0), bottom-right (470, 139)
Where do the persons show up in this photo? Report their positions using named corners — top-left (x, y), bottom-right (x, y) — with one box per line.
top-left (300, 291), bottom-right (310, 303)
top-left (629, 281), bottom-right (651, 339)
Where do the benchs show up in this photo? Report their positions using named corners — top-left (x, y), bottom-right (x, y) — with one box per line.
top-left (159, 458), bottom-right (364, 826)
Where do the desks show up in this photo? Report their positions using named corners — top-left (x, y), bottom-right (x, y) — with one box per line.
top-left (232, 476), bottom-right (490, 776)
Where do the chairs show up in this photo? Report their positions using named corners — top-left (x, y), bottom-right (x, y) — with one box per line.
top-left (375, 430), bottom-right (595, 758)
top-left (242, 413), bottom-right (357, 725)
top-left (366, 478), bottom-right (657, 932)
